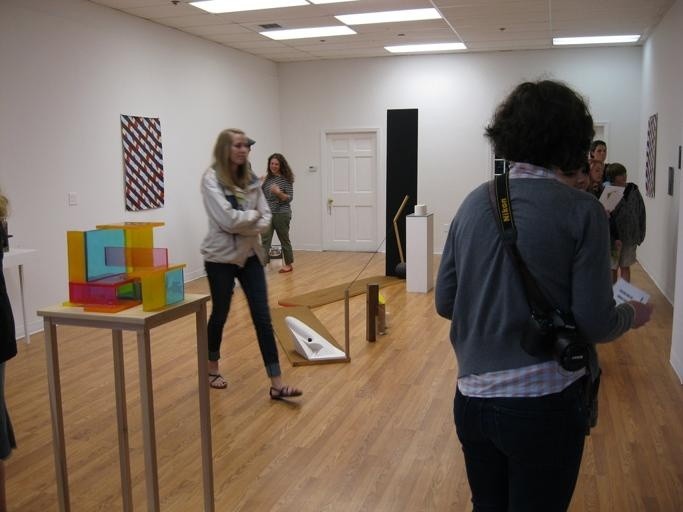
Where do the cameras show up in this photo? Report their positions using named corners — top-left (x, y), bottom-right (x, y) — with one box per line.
top-left (520, 307), bottom-right (592, 371)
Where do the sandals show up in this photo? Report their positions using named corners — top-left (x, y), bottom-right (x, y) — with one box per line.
top-left (209, 373), bottom-right (227, 388)
top-left (270, 385), bottom-right (302, 399)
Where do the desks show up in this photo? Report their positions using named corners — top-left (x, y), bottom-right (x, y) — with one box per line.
top-left (2, 249), bottom-right (34, 344)
top-left (405, 213), bottom-right (434, 293)
top-left (35, 293), bottom-right (215, 511)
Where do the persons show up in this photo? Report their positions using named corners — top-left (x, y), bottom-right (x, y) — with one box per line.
top-left (0, 195), bottom-right (18, 512)
top-left (198, 128), bottom-right (303, 399)
top-left (435, 80), bottom-right (654, 512)
top-left (260, 153), bottom-right (294, 273)
top-left (567, 138), bottom-right (646, 284)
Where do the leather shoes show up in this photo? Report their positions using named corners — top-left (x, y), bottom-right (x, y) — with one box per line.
top-left (279, 267), bottom-right (293, 272)
